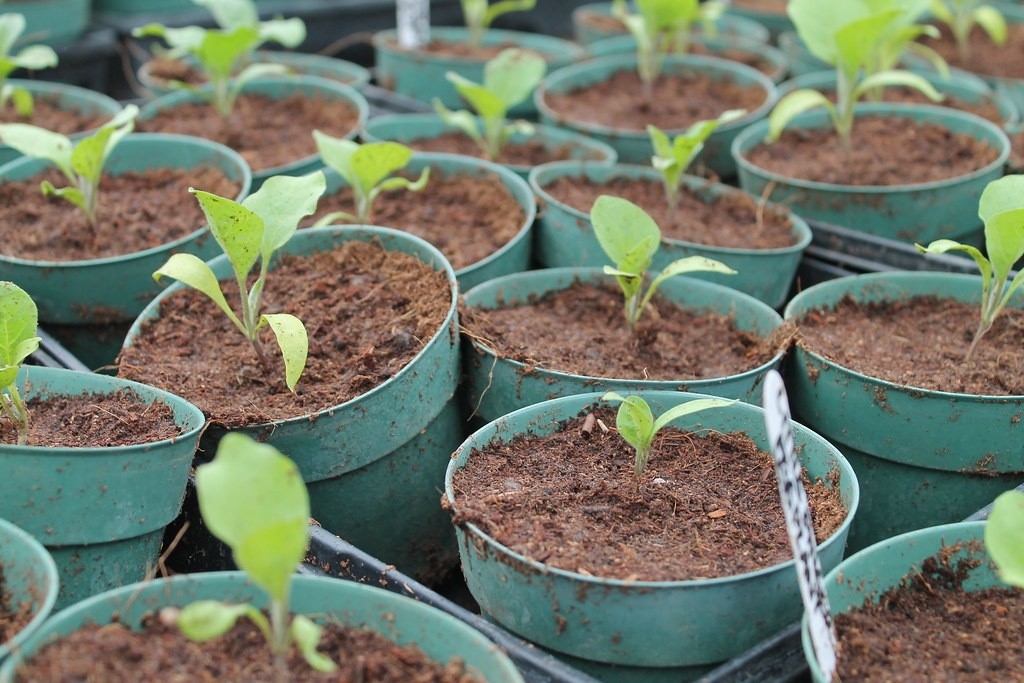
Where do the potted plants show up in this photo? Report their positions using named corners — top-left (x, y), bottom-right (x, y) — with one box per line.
top-left (114, 168), bottom-right (473, 586)
top-left (785, 170), bottom-right (1024, 558)
top-left (0, 0), bottom-right (1024, 376)
top-left (444, 388), bottom-right (858, 683)
top-left (802, 486), bottom-right (1024, 682)
top-left (0, 279), bottom-right (207, 611)
top-left (458, 193), bottom-right (789, 442)
top-left (0, 520), bottom-right (65, 655)
top-left (1, 430), bottom-right (526, 681)
